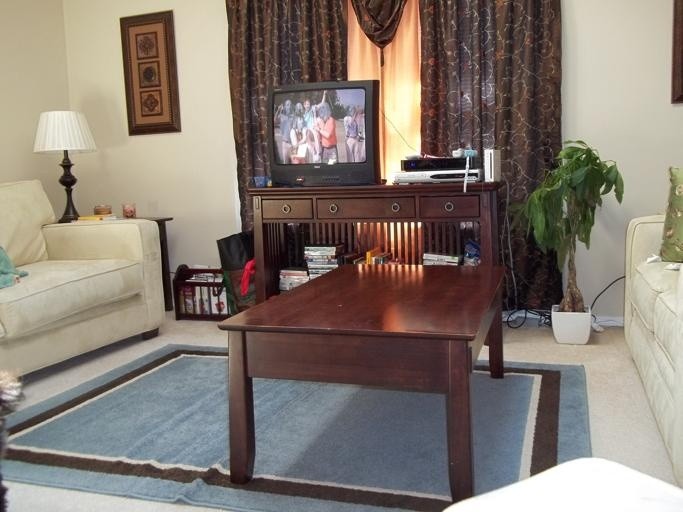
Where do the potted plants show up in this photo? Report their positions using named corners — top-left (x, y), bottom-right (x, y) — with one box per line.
top-left (507, 137), bottom-right (627, 347)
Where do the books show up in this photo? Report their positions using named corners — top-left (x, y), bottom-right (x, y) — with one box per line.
top-left (423, 253), bottom-right (460, 265)
top-left (279, 270), bottom-right (308, 290)
top-left (76, 215), bottom-right (116, 221)
top-left (304, 246), bottom-right (344, 278)
top-left (341, 246), bottom-right (407, 265)
top-left (178, 266), bottom-right (228, 315)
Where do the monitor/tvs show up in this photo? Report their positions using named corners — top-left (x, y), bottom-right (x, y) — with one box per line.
top-left (266, 80), bottom-right (380, 186)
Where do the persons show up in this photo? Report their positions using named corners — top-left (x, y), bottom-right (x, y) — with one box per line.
top-left (353, 105), bottom-right (366, 162)
top-left (292, 103), bottom-right (317, 165)
top-left (312, 101), bottom-right (339, 163)
top-left (302, 90), bottom-right (331, 129)
top-left (273, 100), bottom-right (296, 165)
top-left (343, 104), bottom-right (362, 162)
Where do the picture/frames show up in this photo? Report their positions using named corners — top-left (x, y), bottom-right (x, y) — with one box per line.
top-left (119, 7), bottom-right (183, 138)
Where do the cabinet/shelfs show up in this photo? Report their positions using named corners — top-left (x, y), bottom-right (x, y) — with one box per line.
top-left (146, 217), bottom-right (174, 312)
top-left (247, 180), bottom-right (507, 380)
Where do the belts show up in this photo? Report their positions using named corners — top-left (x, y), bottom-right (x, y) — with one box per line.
top-left (322, 145), bottom-right (337, 150)
top-left (345, 134), bottom-right (358, 139)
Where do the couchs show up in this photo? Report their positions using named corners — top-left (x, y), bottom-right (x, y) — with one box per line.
top-left (622, 214), bottom-right (682, 488)
top-left (0, 178), bottom-right (168, 381)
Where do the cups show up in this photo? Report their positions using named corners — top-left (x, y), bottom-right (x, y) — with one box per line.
top-left (122, 200), bottom-right (136, 218)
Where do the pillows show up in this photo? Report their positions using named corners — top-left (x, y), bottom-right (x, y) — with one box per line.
top-left (658, 164), bottom-right (683, 265)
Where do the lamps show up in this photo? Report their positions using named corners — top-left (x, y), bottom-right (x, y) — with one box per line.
top-left (31, 108), bottom-right (102, 221)
